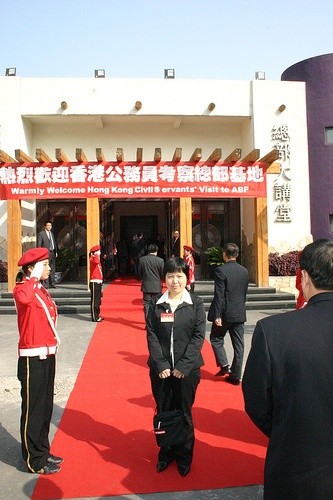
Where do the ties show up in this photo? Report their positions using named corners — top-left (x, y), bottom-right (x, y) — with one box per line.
top-left (48, 232), bottom-right (54, 252)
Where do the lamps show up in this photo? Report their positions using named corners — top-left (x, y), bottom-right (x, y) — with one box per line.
top-left (256, 70), bottom-right (266, 80)
top-left (6, 67), bottom-right (17, 76)
top-left (164, 68), bottom-right (175, 78)
top-left (94, 69), bottom-right (105, 77)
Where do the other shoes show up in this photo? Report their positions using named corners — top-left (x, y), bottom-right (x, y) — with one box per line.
top-left (28, 462), bottom-right (61, 475)
top-left (215, 365), bottom-right (228, 376)
top-left (225, 375), bottom-right (240, 385)
top-left (47, 455), bottom-right (63, 464)
top-left (92, 316), bottom-right (104, 322)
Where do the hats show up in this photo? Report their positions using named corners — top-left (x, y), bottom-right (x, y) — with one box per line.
top-left (17, 248), bottom-right (49, 266)
top-left (183, 246), bottom-right (192, 251)
top-left (90, 246), bottom-right (101, 252)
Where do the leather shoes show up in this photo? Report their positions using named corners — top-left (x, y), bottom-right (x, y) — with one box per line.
top-left (177, 456), bottom-right (190, 477)
top-left (157, 461), bottom-right (168, 472)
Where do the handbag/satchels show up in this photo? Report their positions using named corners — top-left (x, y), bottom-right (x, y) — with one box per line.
top-left (152, 371), bottom-right (192, 448)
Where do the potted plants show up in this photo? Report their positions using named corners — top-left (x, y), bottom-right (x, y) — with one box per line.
top-left (54, 248), bottom-right (77, 284)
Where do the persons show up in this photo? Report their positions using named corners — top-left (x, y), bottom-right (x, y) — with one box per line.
top-left (170, 230), bottom-right (180, 260)
top-left (36, 221), bottom-right (58, 289)
top-left (182, 245), bottom-right (196, 293)
top-left (137, 245), bottom-right (164, 330)
top-left (145, 258), bottom-right (206, 477)
top-left (114, 231), bottom-right (166, 275)
top-left (89, 245), bottom-right (104, 321)
top-left (13, 247), bottom-right (64, 474)
top-left (241, 239), bottom-right (333, 500)
top-left (207, 243), bottom-right (248, 385)
